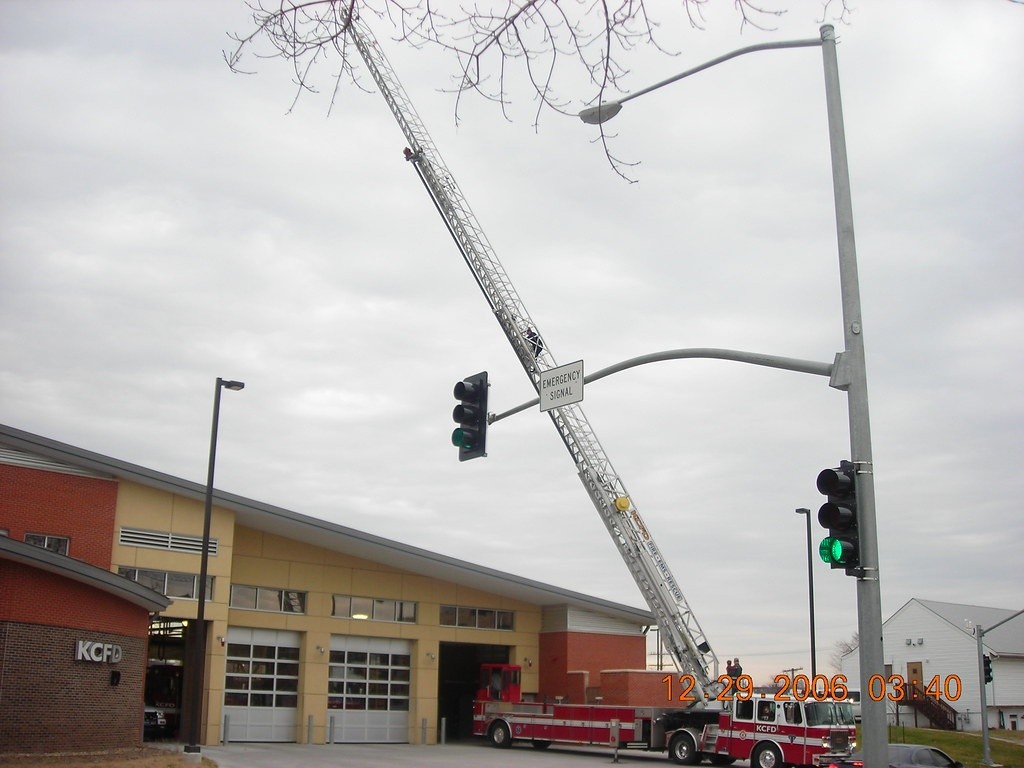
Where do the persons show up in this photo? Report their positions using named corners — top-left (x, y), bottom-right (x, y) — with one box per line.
top-left (726, 659), bottom-right (742, 692)
top-left (763, 706), bottom-right (773, 721)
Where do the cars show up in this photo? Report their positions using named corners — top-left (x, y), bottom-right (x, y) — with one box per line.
top-left (829, 743), bottom-right (964, 768)
top-left (143, 687), bottom-right (181, 740)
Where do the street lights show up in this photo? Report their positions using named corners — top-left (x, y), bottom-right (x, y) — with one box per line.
top-left (795, 508), bottom-right (817, 698)
top-left (579, 25), bottom-right (889, 768)
top-left (184, 377), bottom-right (245, 753)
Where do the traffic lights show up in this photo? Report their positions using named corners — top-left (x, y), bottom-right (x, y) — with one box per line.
top-left (983, 654), bottom-right (993, 684)
top-left (451, 371), bottom-right (488, 462)
top-left (817, 460), bottom-right (860, 570)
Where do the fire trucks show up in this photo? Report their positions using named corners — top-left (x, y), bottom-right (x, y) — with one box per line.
top-left (340, 9), bottom-right (857, 768)
